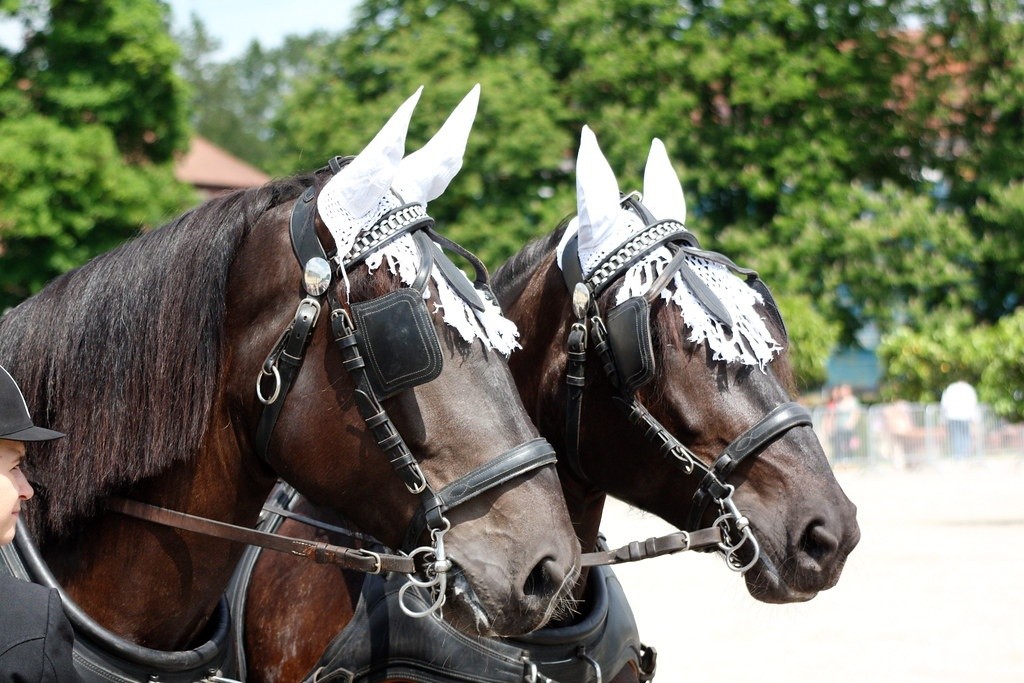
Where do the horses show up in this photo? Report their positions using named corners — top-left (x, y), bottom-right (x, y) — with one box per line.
top-left (209, 118), bottom-right (862, 683)
top-left (0, 84), bottom-right (582, 680)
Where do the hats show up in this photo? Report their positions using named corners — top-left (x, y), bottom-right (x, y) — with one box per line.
top-left (1, 364), bottom-right (65, 440)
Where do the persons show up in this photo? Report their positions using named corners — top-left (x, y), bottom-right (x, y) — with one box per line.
top-left (798, 372), bottom-right (980, 472)
top-left (0, 367), bottom-right (84, 683)
